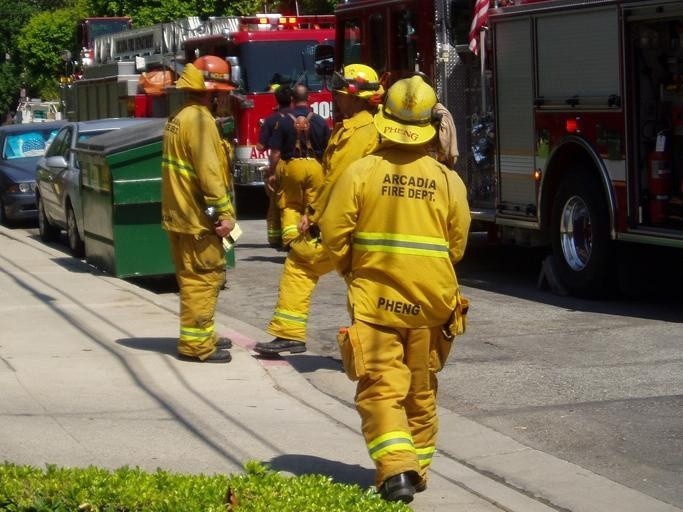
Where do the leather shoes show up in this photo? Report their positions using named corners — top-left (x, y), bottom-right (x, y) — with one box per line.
top-left (180, 348), bottom-right (231, 363)
top-left (215, 338), bottom-right (232, 348)
top-left (378, 472), bottom-right (425, 504)
top-left (254, 337), bottom-right (306, 354)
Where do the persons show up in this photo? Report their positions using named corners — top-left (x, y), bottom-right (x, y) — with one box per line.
top-left (317, 73), bottom-right (473, 504)
top-left (162, 55), bottom-right (236, 361)
top-left (255, 88), bottom-right (290, 246)
top-left (252, 61), bottom-right (384, 356)
top-left (270, 85), bottom-right (330, 243)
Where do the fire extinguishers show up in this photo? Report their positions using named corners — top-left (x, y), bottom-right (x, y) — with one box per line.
top-left (646, 128), bottom-right (671, 224)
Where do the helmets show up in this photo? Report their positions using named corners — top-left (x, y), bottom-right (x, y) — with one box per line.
top-left (374, 76), bottom-right (439, 145)
top-left (177, 55), bottom-right (239, 92)
top-left (336, 64), bottom-right (384, 98)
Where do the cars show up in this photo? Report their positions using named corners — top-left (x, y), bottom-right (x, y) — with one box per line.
top-left (0, 118), bottom-right (73, 229)
top-left (32, 115), bottom-right (168, 260)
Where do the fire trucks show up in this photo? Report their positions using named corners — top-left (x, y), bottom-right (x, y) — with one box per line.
top-left (56, 0), bottom-right (338, 217)
top-left (329, 0), bottom-right (683, 301)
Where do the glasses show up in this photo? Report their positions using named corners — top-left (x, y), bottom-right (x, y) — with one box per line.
top-left (333, 70), bottom-right (378, 90)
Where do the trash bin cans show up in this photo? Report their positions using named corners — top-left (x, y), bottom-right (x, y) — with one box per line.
top-left (71, 116), bottom-right (235, 290)
